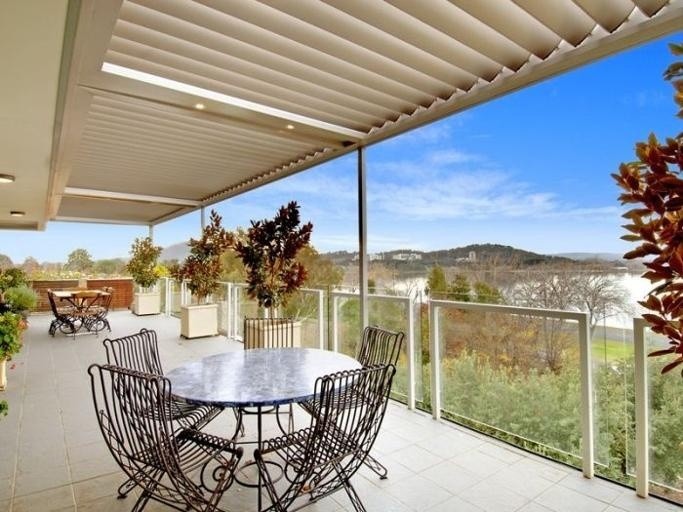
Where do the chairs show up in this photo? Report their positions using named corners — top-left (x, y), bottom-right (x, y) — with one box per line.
top-left (233, 317), bottom-right (296, 445)
top-left (46, 286), bottom-right (114, 340)
top-left (252, 363), bottom-right (397, 511)
top-left (85, 363), bottom-right (244, 511)
top-left (297, 324), bottom-right (406, 490)
top-left (103, 327), bottom-right (227, 470)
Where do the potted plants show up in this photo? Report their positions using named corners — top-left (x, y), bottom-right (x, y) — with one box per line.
top-left (0, 312), bottom-right (29, 391)
top-left (125, 235), bottom-right (167, 316)
top-left (179, 199), bottom-right (314, 349)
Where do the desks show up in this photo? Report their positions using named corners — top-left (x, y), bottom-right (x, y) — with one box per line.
top-left (157, 345), bottom-right (364, 489)
